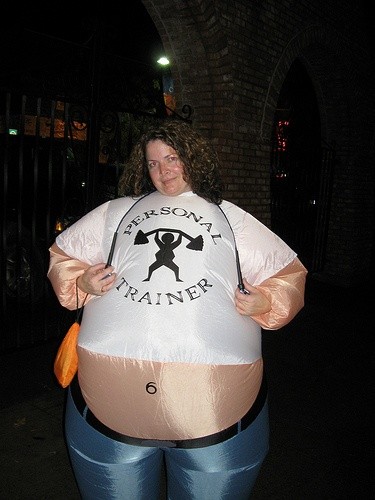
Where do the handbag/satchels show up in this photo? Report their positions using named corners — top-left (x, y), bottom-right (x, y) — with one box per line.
top-left (53, 276), bottom-right (90, 390)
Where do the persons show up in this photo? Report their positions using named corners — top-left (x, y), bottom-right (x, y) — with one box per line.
top-left (47, 122), bottom-right (308, 500)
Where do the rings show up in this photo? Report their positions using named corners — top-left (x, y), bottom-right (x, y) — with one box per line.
top-left (101, 287), bottom-right (105, 293)
